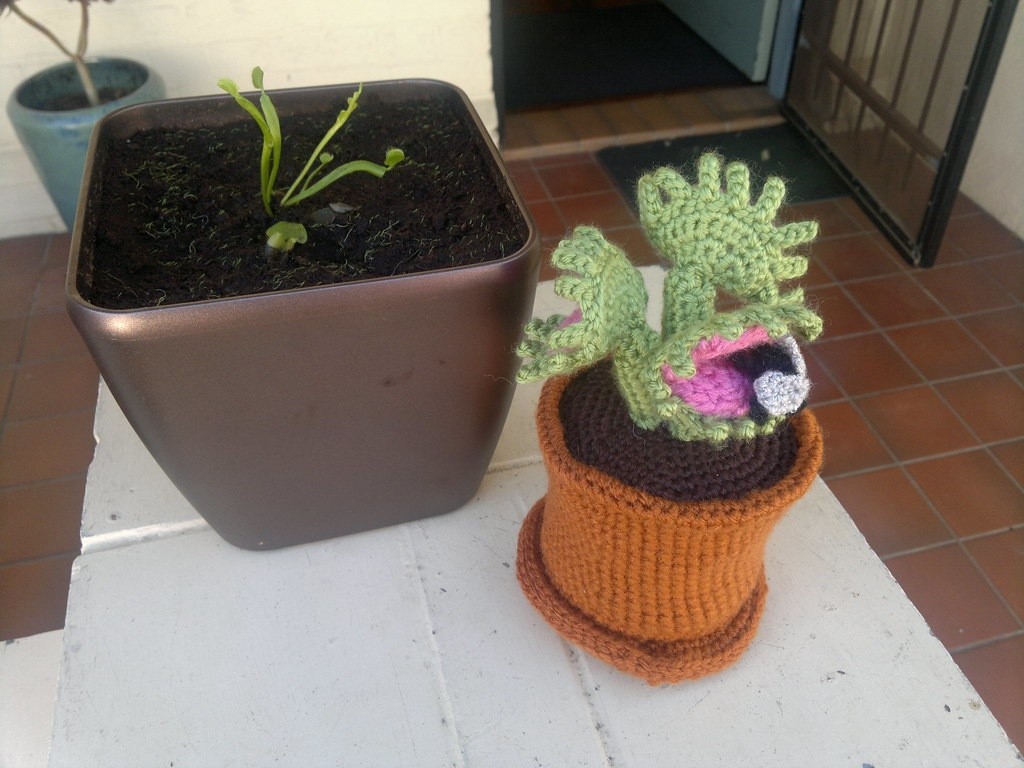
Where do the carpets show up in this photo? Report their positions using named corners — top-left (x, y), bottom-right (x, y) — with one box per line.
top-left (590, 119), bottom-right (858, 234)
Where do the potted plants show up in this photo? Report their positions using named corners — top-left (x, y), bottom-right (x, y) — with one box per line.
top-left (0, 0), bottom-right (167, 241)
top-left (65, 57), bottom-right (537, 548)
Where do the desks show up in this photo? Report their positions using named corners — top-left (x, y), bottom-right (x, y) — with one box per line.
top-left (58, 263), bottom-right (1024, 768)
